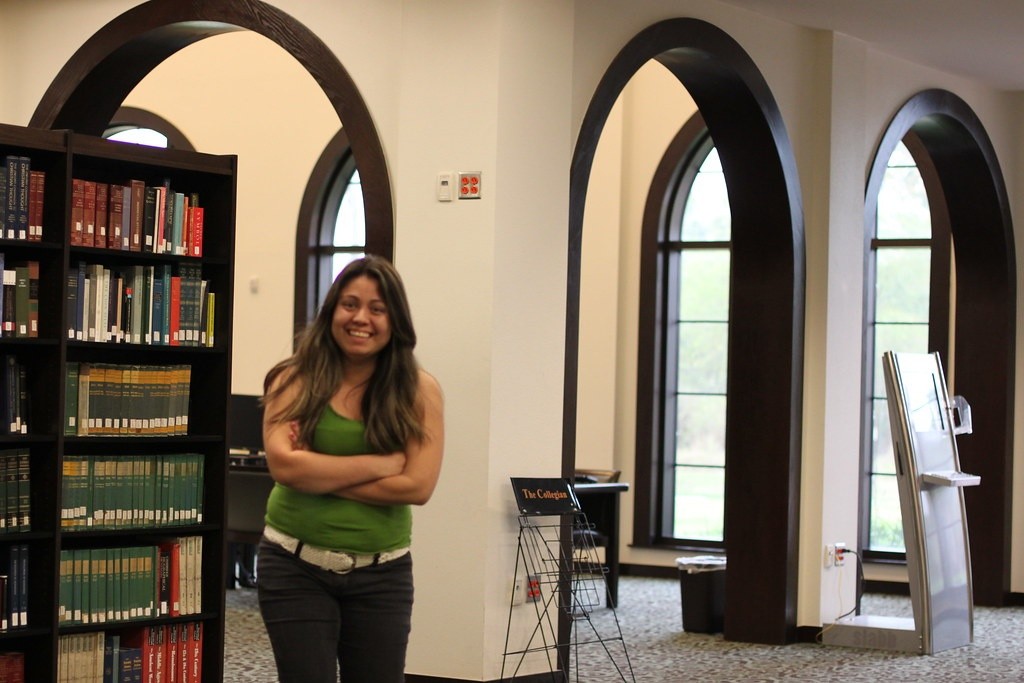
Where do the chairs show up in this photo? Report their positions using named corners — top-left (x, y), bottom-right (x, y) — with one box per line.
top-left (574, 468), bottom-right (621, 483)
top-left (225, 469), bottom-right (274, 589)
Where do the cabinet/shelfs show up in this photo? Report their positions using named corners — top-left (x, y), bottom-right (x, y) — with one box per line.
top-left (0, 125), bottom-right (239, 682)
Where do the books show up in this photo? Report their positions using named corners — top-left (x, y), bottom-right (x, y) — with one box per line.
top-left (63, 355), bottom-right (193, 437)
top-left (229, 448), bottom-right (250, 455)
top-left (58, 618), bottom-right (203, 683)
top-left (59, 533), bottom-right (203, 626)
top-left (0, 542), bottom-right (34, 632)
top-left (62, 449), bottom-right (207, 528)
top-left (0, 249), bottom-right (40, 339)
top-left (0, 447), bottom-right (32, 533)
top-left (68, 168), bottom-right (209, 257)
top-left (0, 648), bottom-right (30, 683)
top-left (63, 253), bottom-right (215, 346)
top-left (0, 151), bottom-right (47, 242)
top-left (0, 349), bottom-right (31, 434)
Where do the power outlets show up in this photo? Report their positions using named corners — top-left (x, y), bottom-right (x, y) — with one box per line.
top-left (834, 542), bottom-right (846, 566)
top-left (525, 575), bottom-right (541, 602)
top-left (512, 578), bottom-right (523, 606)
top-left (825, 544), bottom-right (835, 568)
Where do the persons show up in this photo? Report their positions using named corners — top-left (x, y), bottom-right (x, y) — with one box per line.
top-left (255, 253), bottom-right (445, 683)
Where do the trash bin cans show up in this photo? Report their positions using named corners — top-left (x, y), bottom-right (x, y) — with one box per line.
top-left (675, 555), bottom-right (727, 634)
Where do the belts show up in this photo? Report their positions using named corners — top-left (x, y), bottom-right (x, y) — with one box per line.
top-left (263, 527), bottom-right (411, 572)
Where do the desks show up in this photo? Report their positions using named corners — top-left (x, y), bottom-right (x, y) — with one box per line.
top-left (573, 483), bottom-right (630, 610)
